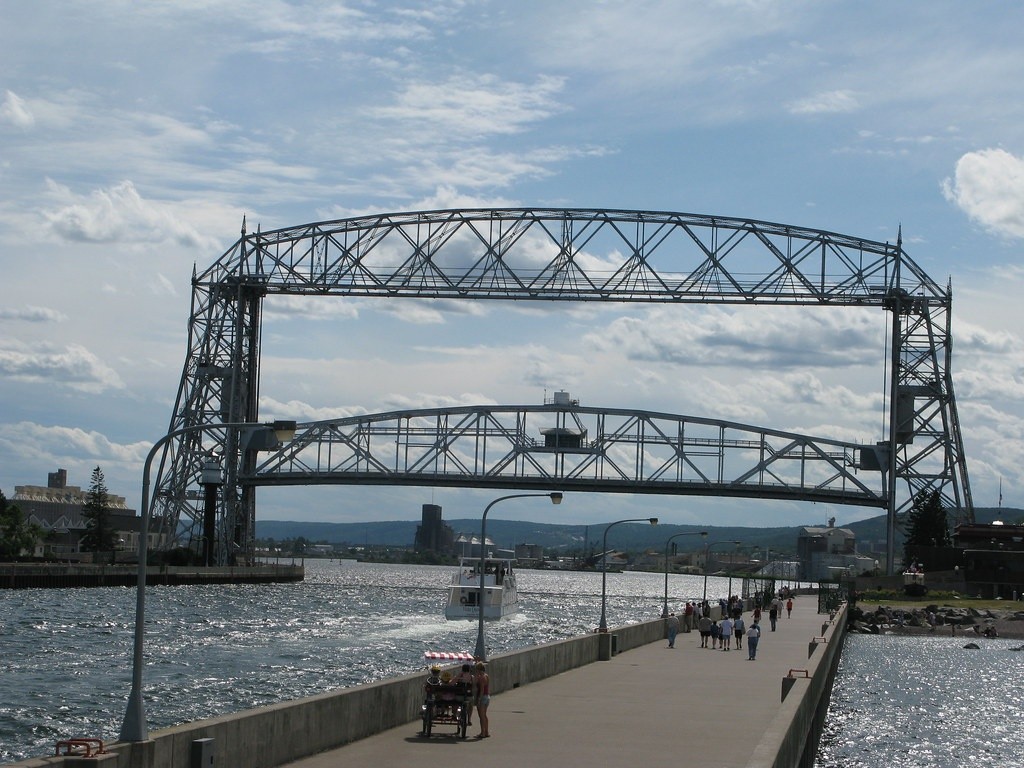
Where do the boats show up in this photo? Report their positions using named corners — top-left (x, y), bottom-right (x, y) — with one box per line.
top-left (444, 536), bottom-right (518, 622)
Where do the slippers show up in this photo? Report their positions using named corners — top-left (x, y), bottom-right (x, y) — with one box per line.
top-left (476, 733), bottom-right (490, 739)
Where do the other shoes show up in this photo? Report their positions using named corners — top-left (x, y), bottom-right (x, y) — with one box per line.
top-left (702, 642), bottom-right (704, 648)
top-left (720, 645), bottom-right (722, 648)
top-left (749, 657), bottom-right (751, 659)
top-left (724, 648), bottom-right (726, 651)
top-left (669, 643), bottom-right (672, 647)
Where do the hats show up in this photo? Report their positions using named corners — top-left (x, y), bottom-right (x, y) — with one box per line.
top-left (462, 664), bottom-right (472, 672)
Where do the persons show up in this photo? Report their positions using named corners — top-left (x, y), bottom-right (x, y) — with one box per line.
top-left (699, 612), bottom-right (712, 648)
top-left (427, 662), bottom-right (474, 726)
top-left (751, 604), bottom-right (761, 623)
top-left (721, 615), bottom-right (733, 651)
top-left (769, 605), bottom-right (777, 631)
top-left (755, 583), bottom-right (775, 610)
top-left (787, 599), bottom-right (792, 619)
top-left (692, 601), bottom-right (710, 629)
top-left (711, 621), bottom-right (719, 649)
top-left (750, 620), bottom-right (760, 636)
top-left (684, 603), bottom-right (693, 633)
top-left (779, 586), bottom-right (791, 597)
top-left (719, 623), bottom-right (723, 648)
top-left (734, 616), bottom-right (746, 649)
top-left (719, 595), bottom-right (743, 617)
top-left (475, 662), bottom-right (490, 738)
top-left (667, 612), bottom-right (679, 647)
top-left (486, 565), bottom-right (512, 585)
top-left (771, 597), bottom-right (784, 617)
top-left (745, 625), bottom-right (759, 660)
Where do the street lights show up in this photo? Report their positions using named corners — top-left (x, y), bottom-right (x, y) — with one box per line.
top-left (473, 491), bottom-right (565, 662)
top-left (116, 418), bottom-right (300, 742)
top-left (599, 517), bottom-right (659, 631)
top-left (703, 540), bottom-right (740, 601)
top-left (661, 532), bottom-right (708, 618)
top-left (728, 545), bottom-right (801, 597)
